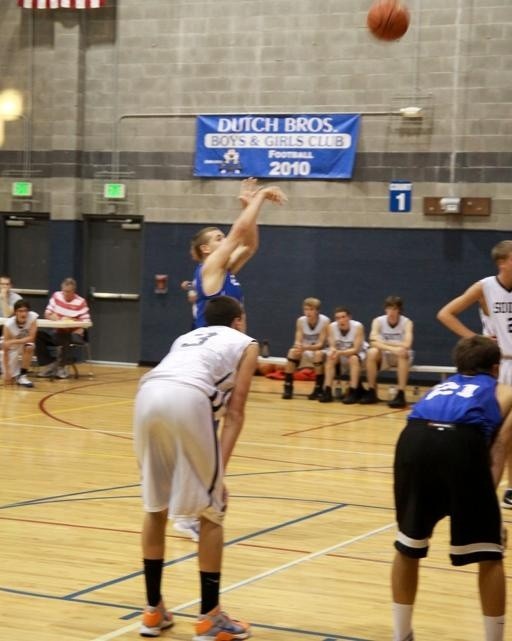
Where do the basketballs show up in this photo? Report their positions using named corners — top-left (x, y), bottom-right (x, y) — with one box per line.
top-left (368, 0), bottom-right (411, 42)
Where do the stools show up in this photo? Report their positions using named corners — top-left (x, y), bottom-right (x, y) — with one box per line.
top-left (60, 344), bottom-right (82, 379)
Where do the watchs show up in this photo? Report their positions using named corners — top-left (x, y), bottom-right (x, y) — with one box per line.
top-left (188, 281), bottom-right (192, 289)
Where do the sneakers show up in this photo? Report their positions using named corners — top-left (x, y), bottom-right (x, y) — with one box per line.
top-left (37, 368), bottom-right (54, 376)
top-left (359, 391), bottom-right (377, 402)
top-left (56, 367), bottom-right (68, 378)
top-left (141, 597), bottom-right (175, 635)
top-left (342, 389), bottom-right (357, 402)
top-left (15, 376), bottom-right (31, 385)
top-left (499, 489), bottom-right (512, 509)
top-left (196, 606), bottom-right (249, 636)
top-left (321, 392), bottom-right (332, 402)
top-left (390, 398), bottom-right (408, 408)
top-left (307, 387), bottom-right (322, 399)
top-left (283, 384), bottom-right (292, 399)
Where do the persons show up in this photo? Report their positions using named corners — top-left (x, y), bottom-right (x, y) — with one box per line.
top-left (36, 277), bottom-right (91, 379)
top-left (0, 275), bottom-right (34, 376)
top-left (181, 238), bottom-right (203, 329)
top-left (392, 336), bottom-right (512, 641)
top-left (3, 300), bottom-right (39, 387)
top-left (435, 239), bottom-right (512, 508)
top-left (133, 295), bottom-right (260, 641)
top-left (359, 294), bottom-right (415, 406)
top-left (192, 177), bottom-right (289, 330)
top-left (319, 306), bottom-right (368, 404)
top-left (282, 297), bottom-right (329, 400)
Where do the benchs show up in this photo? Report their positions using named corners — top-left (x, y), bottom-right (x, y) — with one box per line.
top-left (257, 355), bottom-right (458, 380)
